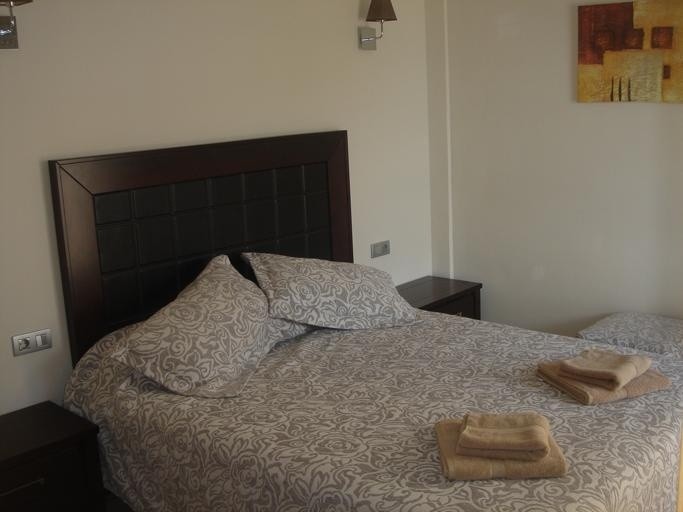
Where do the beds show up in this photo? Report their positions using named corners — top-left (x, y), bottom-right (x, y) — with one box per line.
top-left (47, 130), bottom-right (683, 512)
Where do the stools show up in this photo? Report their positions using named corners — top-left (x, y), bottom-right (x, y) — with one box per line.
top-left (576, 311), bottom-right (683, 356)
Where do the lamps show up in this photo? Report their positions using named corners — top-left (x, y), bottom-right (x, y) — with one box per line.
top-left (358, 1), bottom-right (398, 50)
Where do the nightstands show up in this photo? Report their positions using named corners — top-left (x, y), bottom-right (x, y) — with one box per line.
top-left (2, 399), bottom-right (109, 512)
top-left (395, 274), bottom-right (484, 322)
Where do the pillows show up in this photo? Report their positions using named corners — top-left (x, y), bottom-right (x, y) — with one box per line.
top-left (112, 254), bottom-right (314, 396)
top-left (240, 252), bottom-right (426, 331)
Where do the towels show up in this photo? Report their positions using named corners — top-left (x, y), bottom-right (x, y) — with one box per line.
top-left (431, 347), bottom-right (673, 480)
